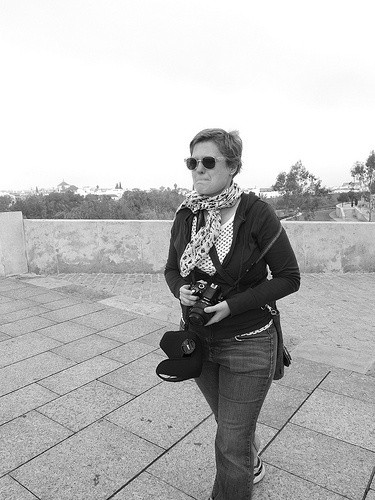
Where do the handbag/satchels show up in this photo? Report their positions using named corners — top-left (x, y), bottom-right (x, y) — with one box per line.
top-left (271, 306), bottom-right (291, 379)
top-left (156, 330), bottom-right (204, 383)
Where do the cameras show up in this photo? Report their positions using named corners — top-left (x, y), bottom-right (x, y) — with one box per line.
top-left (187, 281), bottom-right (224, 329)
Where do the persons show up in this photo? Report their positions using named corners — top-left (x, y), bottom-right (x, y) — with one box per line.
top-left (163, 127), bottom-right (302, 500)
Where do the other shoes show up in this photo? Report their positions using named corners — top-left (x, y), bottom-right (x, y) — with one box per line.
top-left (253, 455), bottom-right (265, 484)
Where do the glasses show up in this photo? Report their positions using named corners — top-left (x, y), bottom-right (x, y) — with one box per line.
top-left (183, 155), bottom-right (229, 171)
